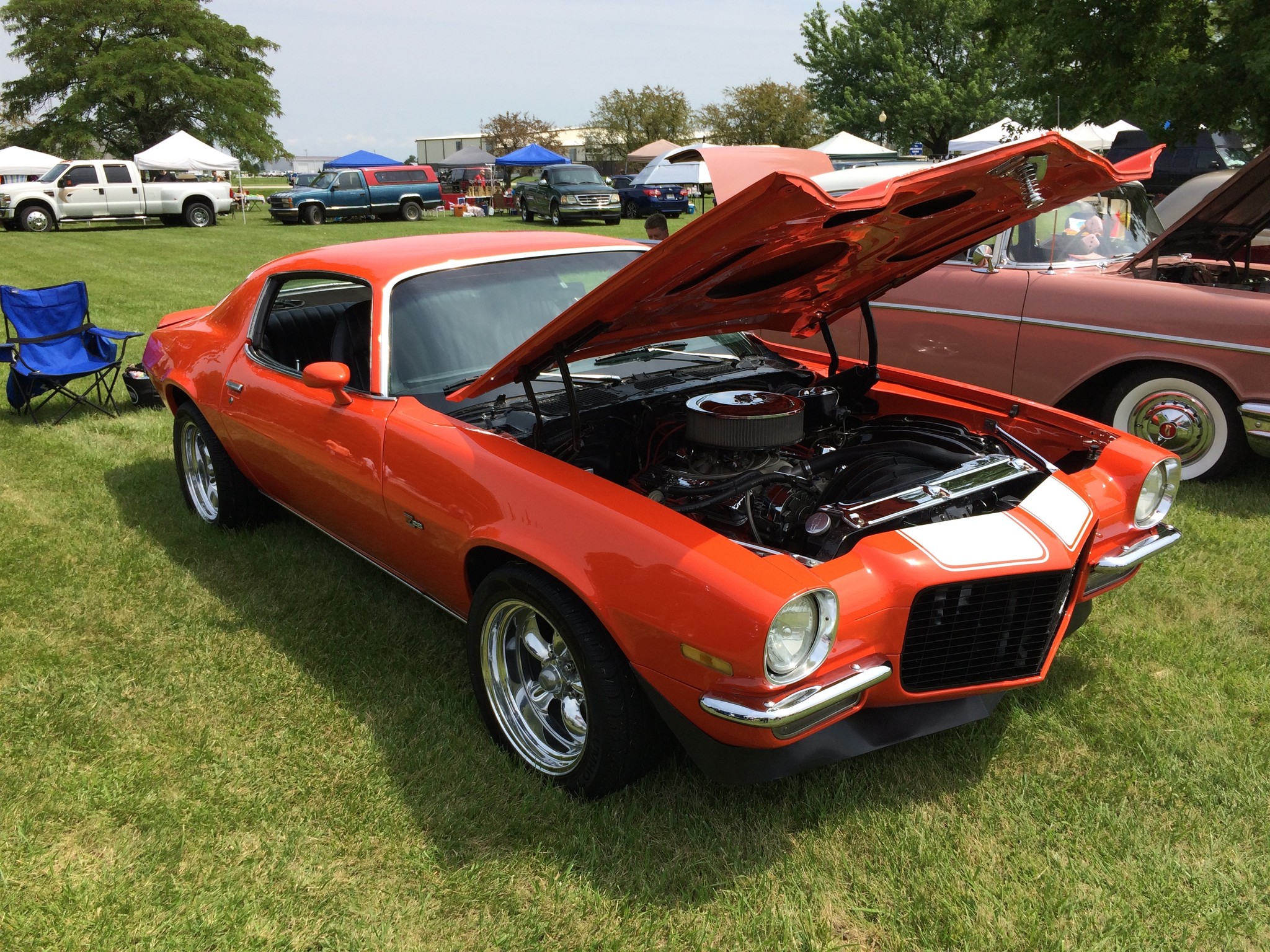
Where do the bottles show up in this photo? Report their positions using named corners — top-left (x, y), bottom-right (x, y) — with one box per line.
top-left (692, 187), bottom-right (696, 193)
top-left (687, 187), bottom-right (691, 194)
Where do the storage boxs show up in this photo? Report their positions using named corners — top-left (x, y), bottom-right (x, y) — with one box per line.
top-left (687, 204), bottom-right (694, 214)
top-left (453, 205), bottom-right (467, 217)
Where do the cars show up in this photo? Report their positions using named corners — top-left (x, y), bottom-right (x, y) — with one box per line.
top-left (288, 172), bottom-right (321, 190)
top-left (606, 174), bottom-right (691, 219)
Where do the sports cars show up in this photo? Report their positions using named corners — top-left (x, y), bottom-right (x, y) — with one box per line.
top-left (663, 144), bottom-right (1270, 487)
top-left (143, 132), bottom-right (1192, 800)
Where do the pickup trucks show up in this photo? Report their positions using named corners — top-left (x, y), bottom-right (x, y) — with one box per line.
top-left (260, 169), bottom-right (295, 177)
top-left (0, 159), bottom-right (240, 233)
top-left (266, 164), bottom-right (447, 226)
top-left (515, 163), bottom-right (623, 227)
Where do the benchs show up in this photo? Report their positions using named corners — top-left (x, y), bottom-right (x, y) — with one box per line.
top-left (265, 301), bottom-right (361, 372)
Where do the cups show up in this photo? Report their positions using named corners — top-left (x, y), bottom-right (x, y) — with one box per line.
top-left (246, 190), bottom-right (248, 195)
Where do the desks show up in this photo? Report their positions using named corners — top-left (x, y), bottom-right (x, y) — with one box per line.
top-left (688, 192), bottom-right (701, 212)
top-left (466, 196), bottom-right (513, 215)
top-left (244, 195), bottom-right (265, 211)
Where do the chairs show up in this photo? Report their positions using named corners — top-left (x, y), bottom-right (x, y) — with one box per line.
top-left (0, 281), bottom-right (145, 429)
top-left (424, 206), bottom-right (445, 218)
top-left (331, 292), bottom-right (461, 393)
top-left (494, 281), bottom-right (562, 359)
top-left (493, 194), bottom-right (513, 216)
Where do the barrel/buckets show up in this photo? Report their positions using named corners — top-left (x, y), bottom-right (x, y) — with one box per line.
top-left (453, 204), bottom-right (466, 216)
top-left (122, 362), bottom-right (167, 409)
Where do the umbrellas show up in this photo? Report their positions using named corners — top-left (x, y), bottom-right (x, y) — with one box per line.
top-left (628, 135), bottom-right (727, 217)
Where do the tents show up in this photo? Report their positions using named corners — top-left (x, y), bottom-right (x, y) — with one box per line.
top-left (323, 150), bottom-right (405, 168)
top-left (495, 143), bottom-right (572, 180)
top-left (807, 131), bottom-right (899, 164)
top-left (0, 145), bottom-right (71, 180)
top-left (437, 144), bottom-right (506, 192)
top-left (625, 139), bottom-right (682, 174)
top-left (946, 114), bottom-right (1145, 158)
top-left (133, 130), bottom-right (246, 225)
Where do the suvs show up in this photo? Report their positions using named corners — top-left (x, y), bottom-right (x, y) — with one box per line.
top-left (440, 167), bottom-right (493, 194)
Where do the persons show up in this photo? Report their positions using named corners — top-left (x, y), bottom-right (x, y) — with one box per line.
top-left (210, 170), bottom-right (217, 182)
top-left (473, 169), bottom-right (486, 205)
top-left (644, 213), bottom-right (669, 242)
top-left (217, 176), bottom-right (233, 216)
top-left (155, 170), bottom-right (174, 182)
top-left (234, 185), bottom-right (245, 204)
